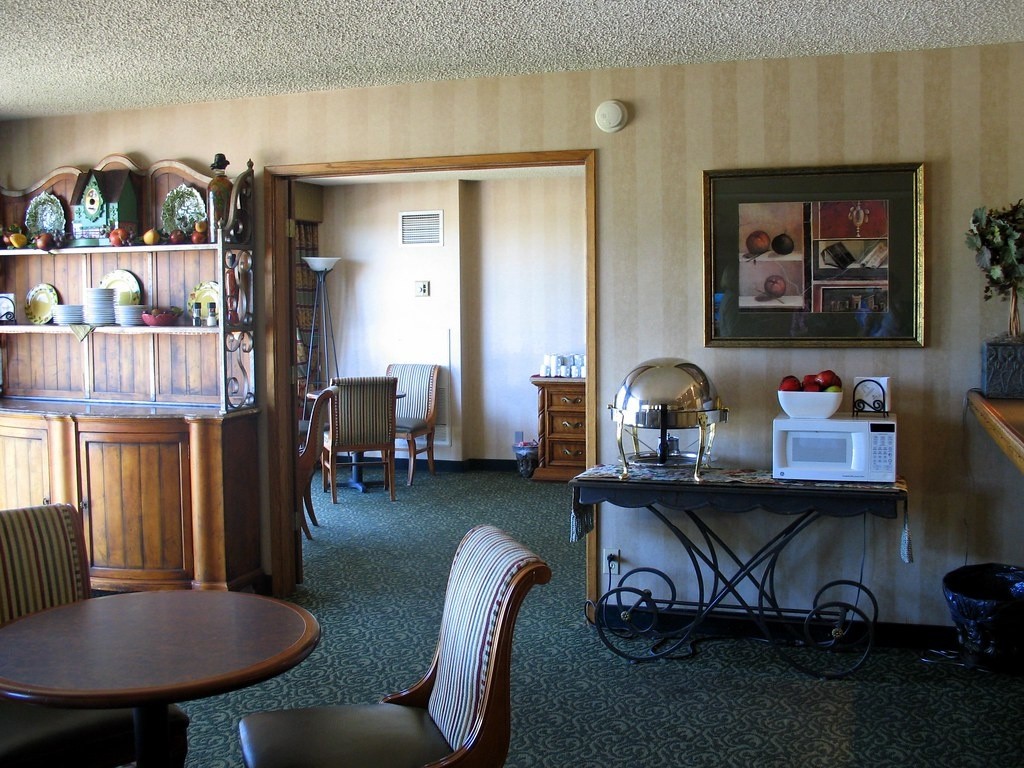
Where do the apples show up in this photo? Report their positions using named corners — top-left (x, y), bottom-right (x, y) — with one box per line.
top-left (170, 220), bottom-right (207, 244)
top-left (110, 227), bottom-right (128, 247)
top-left (35, 232), bottom-right (55, 251)
top-left (779, 369), bottom-right (842, 392)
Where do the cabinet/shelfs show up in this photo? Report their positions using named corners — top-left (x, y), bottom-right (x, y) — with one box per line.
top-left (529, 375), bottom-right (587, 481)
top-left (0, 153), bottom-right (264, 592)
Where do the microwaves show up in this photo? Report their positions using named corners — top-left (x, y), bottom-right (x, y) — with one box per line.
top-left (772, 412), bottom-right (897, 482)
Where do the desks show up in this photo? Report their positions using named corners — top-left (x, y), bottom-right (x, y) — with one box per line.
top-left (568, 462), bottom-right (911, 676)
top-left (306, 390), bottom-right (406, 493)
top-left (965, 391), bottom-right (1024, 476)
top-left (0, 590), bottom-right (321, 768)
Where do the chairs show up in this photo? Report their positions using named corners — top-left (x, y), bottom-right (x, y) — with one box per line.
top-left (297, 365), bottom-right (442, 538)
top-left (239, 526), bottom-right (552, 768)
top-left (0, 503), bottom-right (189, 768)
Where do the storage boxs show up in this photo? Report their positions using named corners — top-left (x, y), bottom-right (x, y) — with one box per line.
top-left (853, 377), bottom-right (892, 411)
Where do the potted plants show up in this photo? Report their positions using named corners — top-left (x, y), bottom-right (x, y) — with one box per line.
top-left (963, 198), bottom-right (1024, 399)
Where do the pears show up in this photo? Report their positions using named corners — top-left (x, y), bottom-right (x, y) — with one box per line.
top-left (151, 305), bottom-right (184, 316)
top-left (9, 234), bottom-right (27, 248)
top-left (142, 227), bottom-right (160, 245)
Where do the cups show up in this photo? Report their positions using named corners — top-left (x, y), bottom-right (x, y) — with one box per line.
top-left (540, 353), bottom-right (586, 378)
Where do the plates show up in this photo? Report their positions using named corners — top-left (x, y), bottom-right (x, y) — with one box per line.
top-left (160, 184), bottom-right (206, 235)
top-left (24, 283), bottom-right (58, 325)
top-left (24, 191), bottom-right (66, 232)
top-left (53, 269), bottom-right (150, 327)
top-left (187, 281), bottom-right (219, 324)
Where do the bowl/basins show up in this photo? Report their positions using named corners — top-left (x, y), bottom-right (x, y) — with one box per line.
top-left (141, 313), bottom-right (178, 327)
top-left (777, 390), bottom-right (844, 420)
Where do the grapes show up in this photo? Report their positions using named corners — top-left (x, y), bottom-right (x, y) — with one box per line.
top-left (121, 230), bottom-right (136, 247)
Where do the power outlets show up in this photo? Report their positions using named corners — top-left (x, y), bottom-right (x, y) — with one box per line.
top-left (603, 548), bottom-right (621, 574)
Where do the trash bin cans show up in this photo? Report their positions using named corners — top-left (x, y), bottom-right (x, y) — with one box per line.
top-left (512, 444), bottom-right (538, 478)
top-left (941, 564), bottom-right (1024, 655)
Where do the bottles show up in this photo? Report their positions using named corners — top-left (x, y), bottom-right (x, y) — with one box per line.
top-left (207, 303), bottom-right (217, 327)
top-left (225, 254), bottom-right (239, 325)
top-left (206, 154), bottom-right (234, 244)
top-left (193, 302), bottom-right (201, 326)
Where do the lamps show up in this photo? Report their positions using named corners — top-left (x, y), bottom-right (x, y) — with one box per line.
top-left (302, 255), bottom-right (340, 423)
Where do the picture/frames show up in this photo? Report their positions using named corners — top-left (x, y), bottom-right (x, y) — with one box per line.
top-left (701, 161), bottom-right (927, 348)
